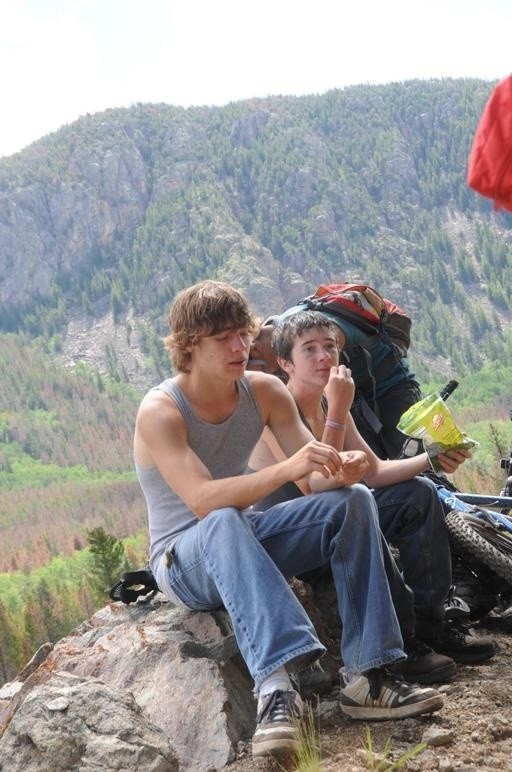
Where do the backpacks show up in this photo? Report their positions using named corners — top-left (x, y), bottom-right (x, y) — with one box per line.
top-left (302, 284), bottom-right (411, 353)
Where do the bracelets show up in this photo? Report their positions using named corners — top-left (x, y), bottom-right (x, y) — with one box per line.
top-left (325, 419), bottom-right (347, 431)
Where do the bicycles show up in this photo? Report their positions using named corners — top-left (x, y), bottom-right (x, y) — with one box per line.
top-left (395, 375), bottom-right (512, 597)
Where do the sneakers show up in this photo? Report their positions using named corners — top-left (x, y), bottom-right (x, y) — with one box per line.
top-left (250, 688), bottom-right (305, 759)
top-left (337, 625), bottom-right (493, 719)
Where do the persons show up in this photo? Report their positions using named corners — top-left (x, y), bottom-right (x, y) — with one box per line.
top-left (133, 279), bottom-right (445, 759)
top-left (248, 309), bottom-right (496, 685)
